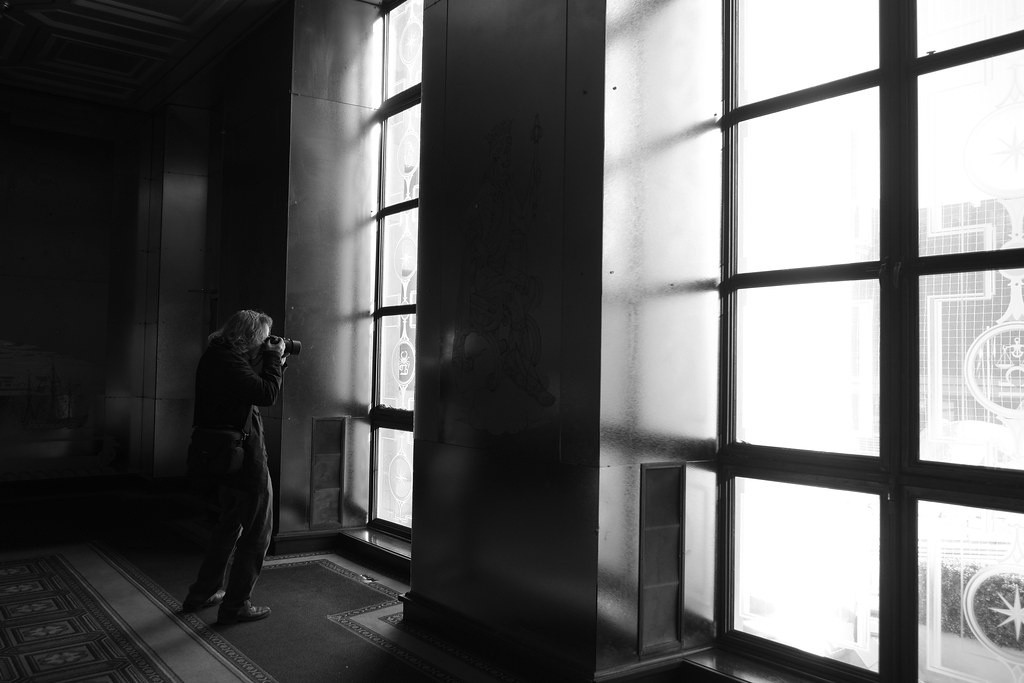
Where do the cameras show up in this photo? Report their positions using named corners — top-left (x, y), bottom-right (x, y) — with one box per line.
top-left (259, 336), bottom-right (302, 357)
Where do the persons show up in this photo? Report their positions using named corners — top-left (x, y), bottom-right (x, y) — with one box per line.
top-left (184, 308), bottom-right (292, 623)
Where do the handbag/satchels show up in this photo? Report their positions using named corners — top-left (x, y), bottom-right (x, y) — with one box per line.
top-left (186, 423), bottom-right (246, 477)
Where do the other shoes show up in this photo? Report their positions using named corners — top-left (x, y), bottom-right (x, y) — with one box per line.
top-left (183, 590), bottom-right (226, 614)
top-left (218, 600), bottom-right (271, 625)
